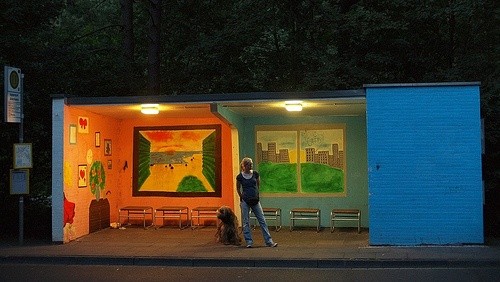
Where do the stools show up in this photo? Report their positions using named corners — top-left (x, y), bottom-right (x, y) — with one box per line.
top-left (154, 206), bottom-right (189, 230)
top-left (249, 208), bottom-right (281, 232)
top-left (289, 208), bottom-right (321, 233)
top-left (190, 207), bottom-right (220, 230)
top-left (118, 205), bottom-right (154, 230)
top-left (330, 209), bottom-right (361, 234)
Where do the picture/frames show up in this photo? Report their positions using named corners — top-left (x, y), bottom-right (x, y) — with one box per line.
top-left (132, 124), bottom-right (223, 198)
top-left (78, 164), bottom-right (88, 188)
top-left (9, 169), bottom-right (29, 195)
top-left (13, 142), bottom-right (32, 169)
top-left (95, 132), bottom-right (100, 147)
top-left (104, 139), bottom-right (112, 156)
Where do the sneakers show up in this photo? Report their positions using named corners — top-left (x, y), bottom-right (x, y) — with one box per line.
top-left (265, 242), bottom-right (277, 247)
top-left (246, 244), bottom-right (252, 248)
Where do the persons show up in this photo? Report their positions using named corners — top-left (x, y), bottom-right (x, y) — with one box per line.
top-left (236, 157), bottom-right (277, 247)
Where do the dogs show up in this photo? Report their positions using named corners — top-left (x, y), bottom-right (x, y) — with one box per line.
top-left (215, 206), bottom-right (242, 246)
